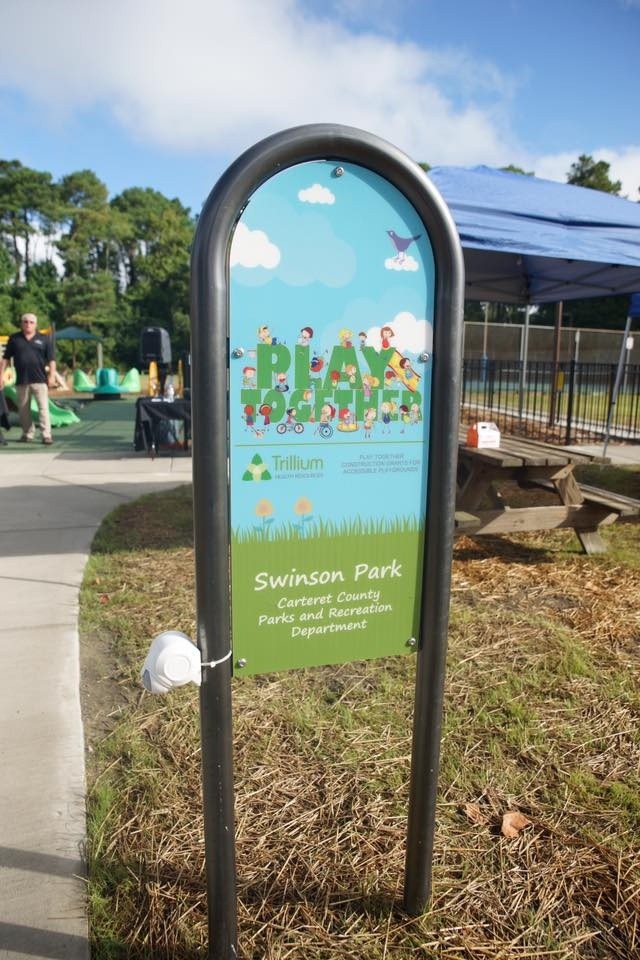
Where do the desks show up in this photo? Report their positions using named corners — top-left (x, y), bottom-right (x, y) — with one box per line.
top-left (458, 422), bottom-right (608, 554)
top-left (136, 396), bottom-right (191, 455)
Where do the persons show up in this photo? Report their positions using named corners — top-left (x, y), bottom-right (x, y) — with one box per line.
top-left (0, 313), bottom-right (56, 444)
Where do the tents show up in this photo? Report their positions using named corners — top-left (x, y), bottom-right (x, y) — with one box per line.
top-left (424, 165), bottom-right (640, 368)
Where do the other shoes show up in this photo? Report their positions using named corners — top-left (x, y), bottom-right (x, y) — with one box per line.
top-left (43, 438), bottom-right (53, 444)
top-left (16, 437), bottom-right (32, 442)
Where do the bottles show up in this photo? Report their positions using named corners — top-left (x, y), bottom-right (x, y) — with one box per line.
top-left (167, 384), bottom-right (174, 403)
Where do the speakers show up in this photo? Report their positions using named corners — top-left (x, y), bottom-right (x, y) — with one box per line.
top-left (139, 630), bottom-right (201, 692)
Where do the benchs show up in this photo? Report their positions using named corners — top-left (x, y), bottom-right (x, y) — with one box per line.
top-left (456, 479), bottom-right (640, 529)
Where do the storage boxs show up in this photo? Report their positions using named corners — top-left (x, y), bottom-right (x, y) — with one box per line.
top-left (466, 421), bottom-right (500, 447)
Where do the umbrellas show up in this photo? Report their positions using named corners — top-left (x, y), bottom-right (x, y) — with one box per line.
top-left (56, 326), bottom-right (96, 368)
